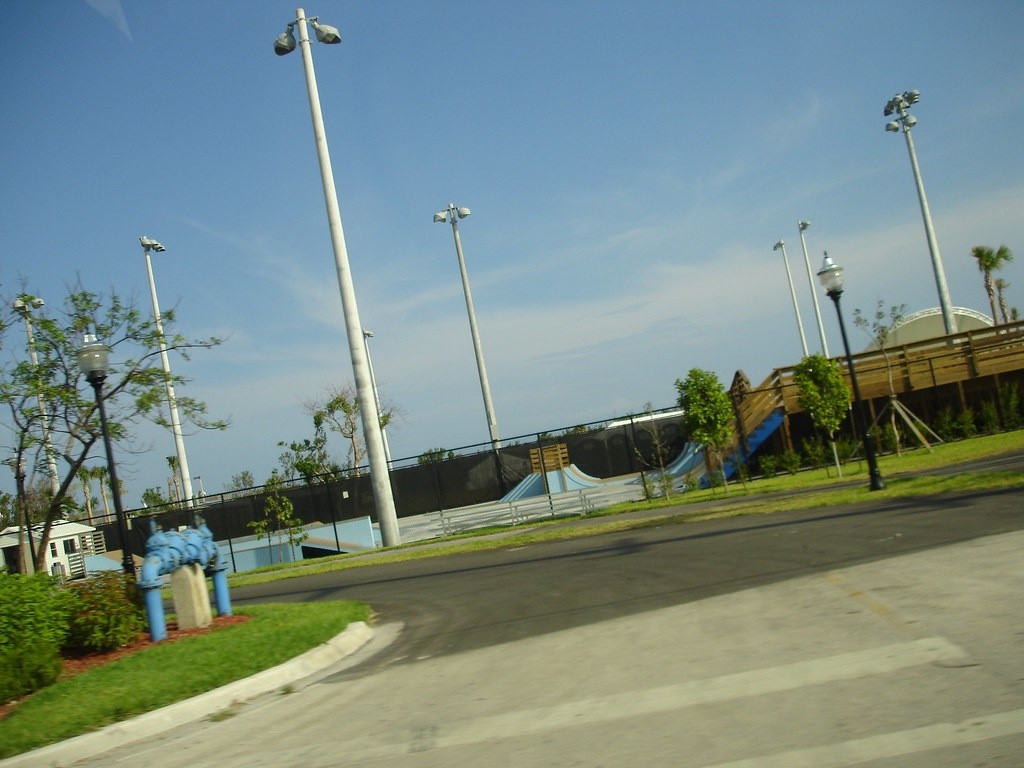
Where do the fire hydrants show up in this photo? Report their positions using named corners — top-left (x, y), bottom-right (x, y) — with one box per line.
top-left (138, 508), bottom-right (234, 643)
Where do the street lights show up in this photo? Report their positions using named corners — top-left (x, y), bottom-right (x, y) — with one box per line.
top-left (140, 235), bottom-right (200, 508)
top-left (818, 253), bottom-right (889, 492)
top-left (9, 447), bottom-right (38, 562)
top-left (774, 241), bottom-right (845, 471)
top-left (274, 7), bottom-right (404, 550)
top-left (10, 297), bottom-right (63, 496)
top-left (74, 323), bottom-right (140, 604)
top-left (794, 219), bottom-right (833, 357)
top-left (432, 204), bottom-right (511, 494)
top-left (883, 88), bottom-right (961, 344)
